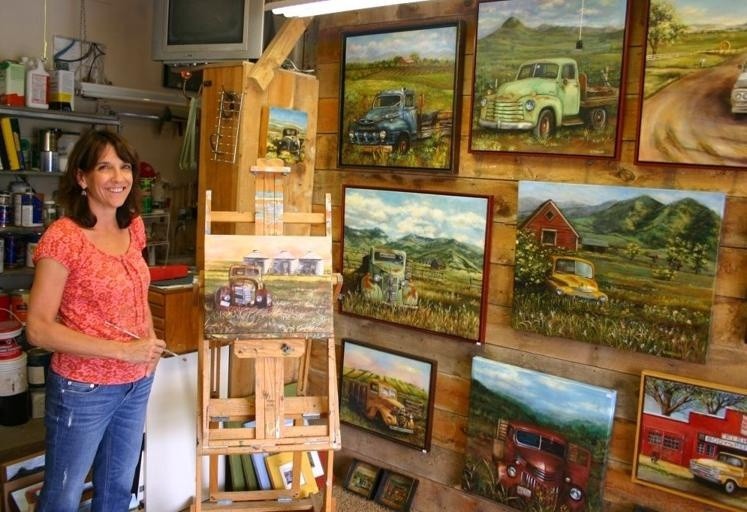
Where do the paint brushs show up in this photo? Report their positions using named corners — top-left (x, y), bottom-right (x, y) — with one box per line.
top-left (104, 321), bottom-right (187, 361)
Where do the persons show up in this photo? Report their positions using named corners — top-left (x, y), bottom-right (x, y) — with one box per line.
top-left (25, 128), bottom-right (167, 512)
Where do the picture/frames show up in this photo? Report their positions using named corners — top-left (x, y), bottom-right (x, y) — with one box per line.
top-left (454, 357), bottom-right (619, 512)
top-left (629, 369), bottom-right (747, 512)
top-left (265, 452), bottom-right (318, 501)
top-left (223, 381), bottom-right (299, 492)
top-left (203, 236), bottom-right (333, 339)
top-left (374, 469), bottom-right (419, 512)
top-left (343, 459), bottom-right (383, 500)
top-left (336, 339), bottom-right (438, 458)
top-left (1, 450), bottom-right (48, 480)
top-left (467, 0), bottom-right (636, 162)
top-left (512, 180), bottom-right (726, 367)
top-left (334, 186), bottom-right (494, 347)
top-left (11, 481), bottom-right (97, 512)
top-left (336, 16), bottom-right (466, 175)
top-left (243, 414), bottom-right (322, 491)
top-left (636, 1), bottom-right (746, 171)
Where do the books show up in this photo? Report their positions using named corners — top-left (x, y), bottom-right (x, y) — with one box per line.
top-left (226, 381), bottom-right (296, 490)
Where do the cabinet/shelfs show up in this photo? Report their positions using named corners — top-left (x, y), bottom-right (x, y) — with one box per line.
top-left (1, 105), bottom-right (170, 275)
top-left (191, 187), bottom-right (343, 512)
top-left (0, 279), bottom-right (145, 458)
top-left (148, 265), bottom-right (201, 357)
top-left (198, 61), bottom-right (320, 273)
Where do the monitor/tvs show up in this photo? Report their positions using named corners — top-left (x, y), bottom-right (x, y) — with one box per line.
top-left (150, 0), bottom-right (264, 63)
